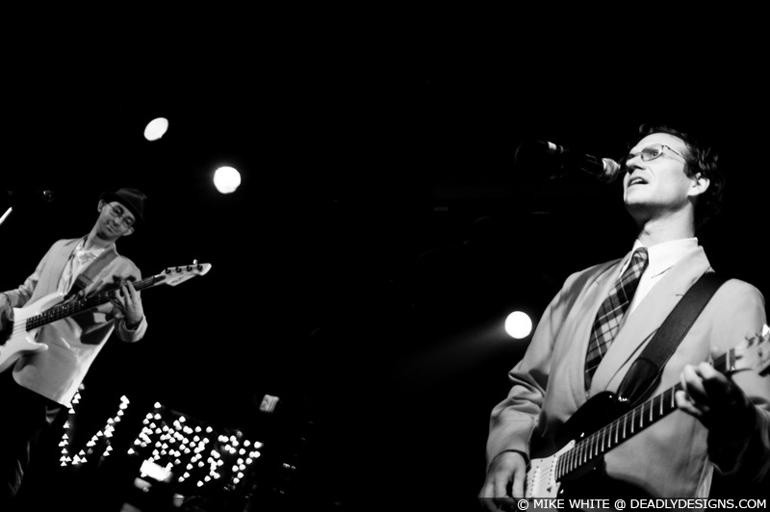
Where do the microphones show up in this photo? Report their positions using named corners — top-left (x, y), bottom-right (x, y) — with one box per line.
top-left (0, 189), bottom-right (54, 202)
top-left (540, 137), bottom-right (621, 182)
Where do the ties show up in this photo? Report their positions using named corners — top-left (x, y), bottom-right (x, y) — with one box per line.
top-left (582, 247), bottom-right (649, 395)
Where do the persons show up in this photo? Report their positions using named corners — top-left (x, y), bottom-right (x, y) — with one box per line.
top-left (0, 187), bottom-right (148, 512)
top-left (479, 123), bottom-right (770, 512)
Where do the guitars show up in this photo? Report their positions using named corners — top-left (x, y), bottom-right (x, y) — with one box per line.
top-left (501, 324), bottom-right (768, 511)
top-left (0, 260), bottom-right (213, 373)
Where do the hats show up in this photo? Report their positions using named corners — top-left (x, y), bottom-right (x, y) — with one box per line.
top-left (109, 190), bottom-right (145, 221)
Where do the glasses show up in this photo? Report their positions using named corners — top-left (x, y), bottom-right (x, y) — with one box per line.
top-left (642, 142), bottom-right (687, 165)
top-left (108, 204), bottom-right (135, 231)
